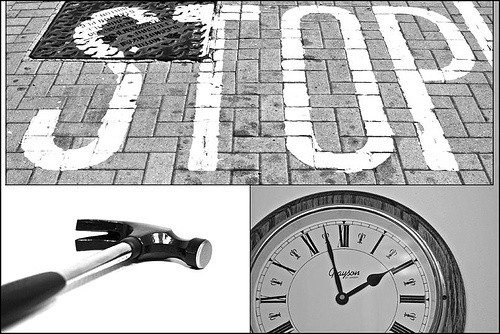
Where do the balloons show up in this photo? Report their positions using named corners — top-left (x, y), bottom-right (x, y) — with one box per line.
top-left (250, 188), bottom-right (466, 333)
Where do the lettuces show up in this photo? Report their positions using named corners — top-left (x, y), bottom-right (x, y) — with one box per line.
top-left (1, 219), bottom-right (213, 333)
